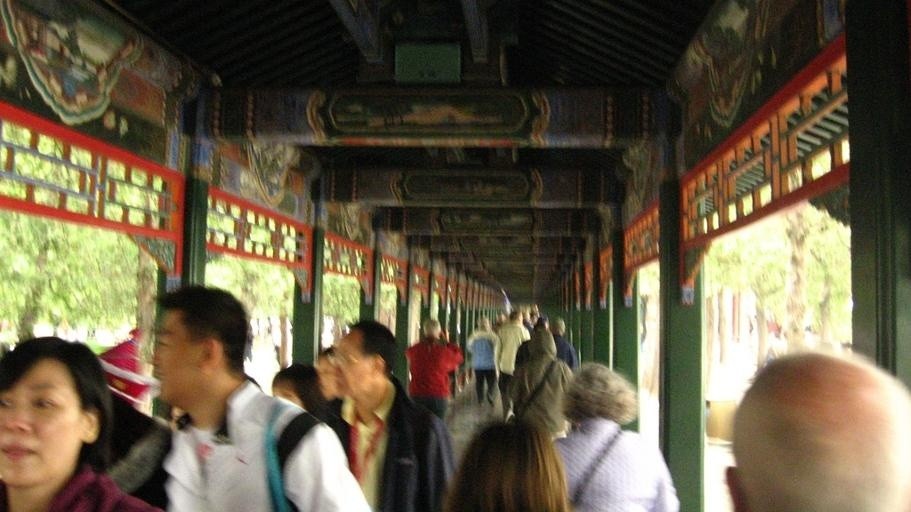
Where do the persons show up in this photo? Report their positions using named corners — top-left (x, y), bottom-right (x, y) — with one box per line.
top-left (725, 349), bottom-right (911, 512)
